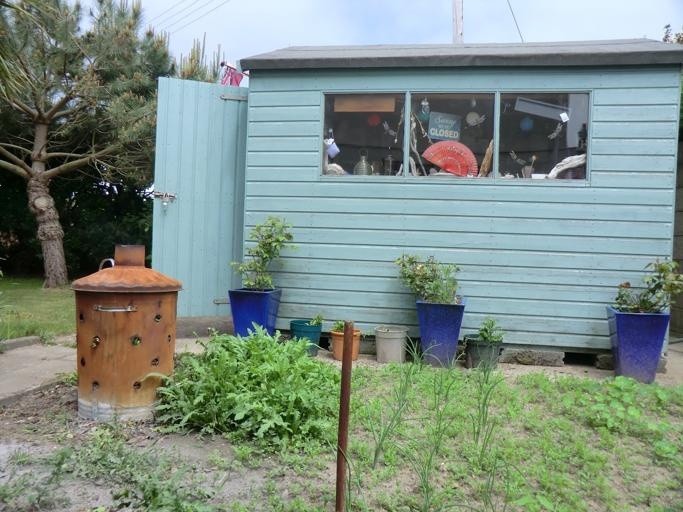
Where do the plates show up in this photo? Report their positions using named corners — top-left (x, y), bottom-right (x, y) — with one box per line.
top-left (532, 173), bottom-right (545, 179)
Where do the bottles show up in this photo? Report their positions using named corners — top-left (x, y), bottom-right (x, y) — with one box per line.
top-left (578, 123), bottom-right (587, 149)
top-left (353, 149), bottom-right (396, 175)
top-left (420, 100), bottom-right (430, 114)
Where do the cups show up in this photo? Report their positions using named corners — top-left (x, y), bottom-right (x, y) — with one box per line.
top-left (521, 166), bottom-right (532, 178)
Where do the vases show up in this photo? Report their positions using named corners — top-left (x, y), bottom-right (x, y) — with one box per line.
top-left (374, 325), bottom-right (410, 363)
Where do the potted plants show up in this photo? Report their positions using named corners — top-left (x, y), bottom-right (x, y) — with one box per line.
top-left (290, 312), bottom-right (324, 357)
top-left (605, 258), bottom-right (683, 384)
top-left (228, 217), bottom-right (297, 337)
top-left (329, 322), bottom-right (361, 361)
top-left (394, 252), bottom-right (466, 369)
top-left (464, 317), bottom-right (507, 370)
